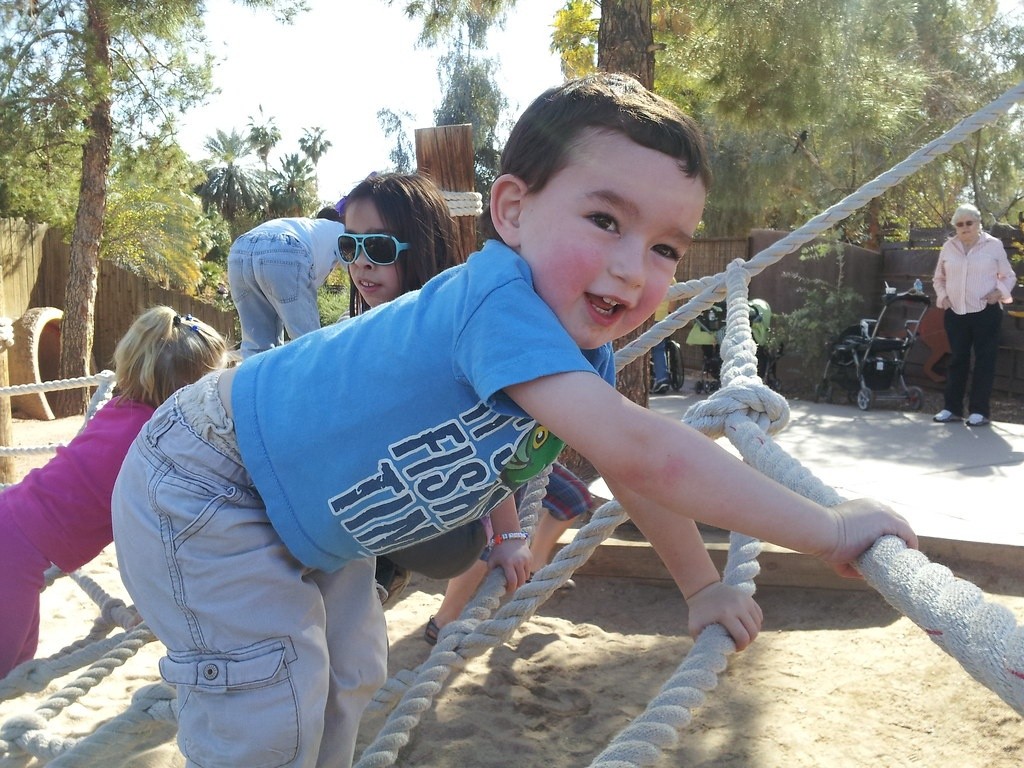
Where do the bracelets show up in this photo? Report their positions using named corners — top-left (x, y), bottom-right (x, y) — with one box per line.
top-left (487, 528), bottom-right (528, 553)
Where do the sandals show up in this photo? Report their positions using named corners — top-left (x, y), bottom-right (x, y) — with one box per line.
top-left (424, 615), bottom-right (461, 652)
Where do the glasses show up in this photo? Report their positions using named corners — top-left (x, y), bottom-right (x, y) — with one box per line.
top-left (337, 234), bottom-right (411, 266)
top-left (955, 221), bottom-right (974, 227)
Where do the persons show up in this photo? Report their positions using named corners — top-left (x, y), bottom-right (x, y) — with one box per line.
top-left (423, 461), bottom-right (591, 648)
top-left (1, 305), bottom-right (229, 682)
top-left (110, 72), bottom-right (921, 768)
top-left (227, 204), bottom-right (347, 363)
top-left (337, 168), bottom-right (535, 601)
top-left (649, 276), bottom-right (678, 394)
top-left (932, 203), bottom-right (1017, 427)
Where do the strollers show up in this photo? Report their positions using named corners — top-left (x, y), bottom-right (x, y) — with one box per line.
top-left (690, 299), bottom-right (785, 398)
top-left (817, 281), bottom-right (933, 410)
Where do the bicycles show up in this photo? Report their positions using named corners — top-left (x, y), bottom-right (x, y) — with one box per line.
top-left (645, 335), bottom-right (686, 391)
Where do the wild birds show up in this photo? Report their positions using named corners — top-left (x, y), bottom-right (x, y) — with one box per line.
top-left (792, 130), bottom-right (809, 155)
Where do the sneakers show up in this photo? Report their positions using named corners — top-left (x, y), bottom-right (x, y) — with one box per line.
top-left (933, 409), bottom-right (962, 422)
top-left (965, 413), bottom-right (990, 426)
top-left (654, 381), bottom-right (670, 393)
